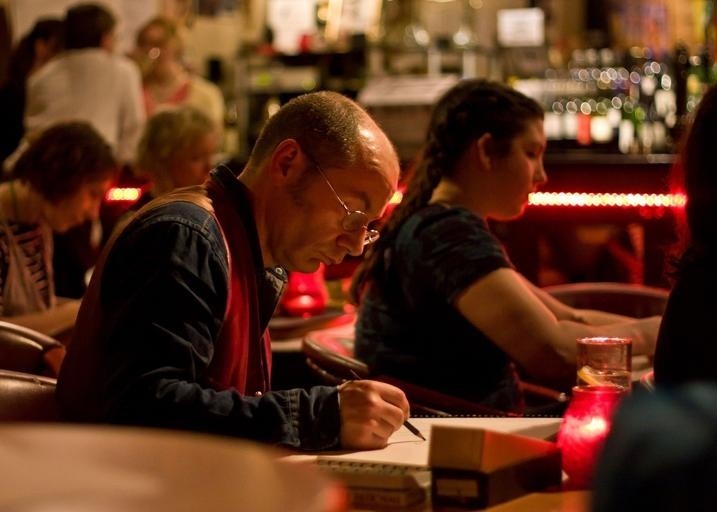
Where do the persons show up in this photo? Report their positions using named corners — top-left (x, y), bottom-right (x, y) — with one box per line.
top-left (0, 1), bottom-right (717, 512)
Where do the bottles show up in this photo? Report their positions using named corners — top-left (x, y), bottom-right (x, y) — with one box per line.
top-left (555, 383), bottom-right (628, 487)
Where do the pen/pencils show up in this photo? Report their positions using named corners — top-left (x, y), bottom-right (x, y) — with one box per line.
top-left (349, 370), bottom-right (427, 442)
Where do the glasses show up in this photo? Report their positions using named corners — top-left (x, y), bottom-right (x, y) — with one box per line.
top-left (299, 149), bottom-right (381, 247)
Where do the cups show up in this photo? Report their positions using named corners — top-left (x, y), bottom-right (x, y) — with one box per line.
top-left (574, 333), bottom-right (634, 370)
